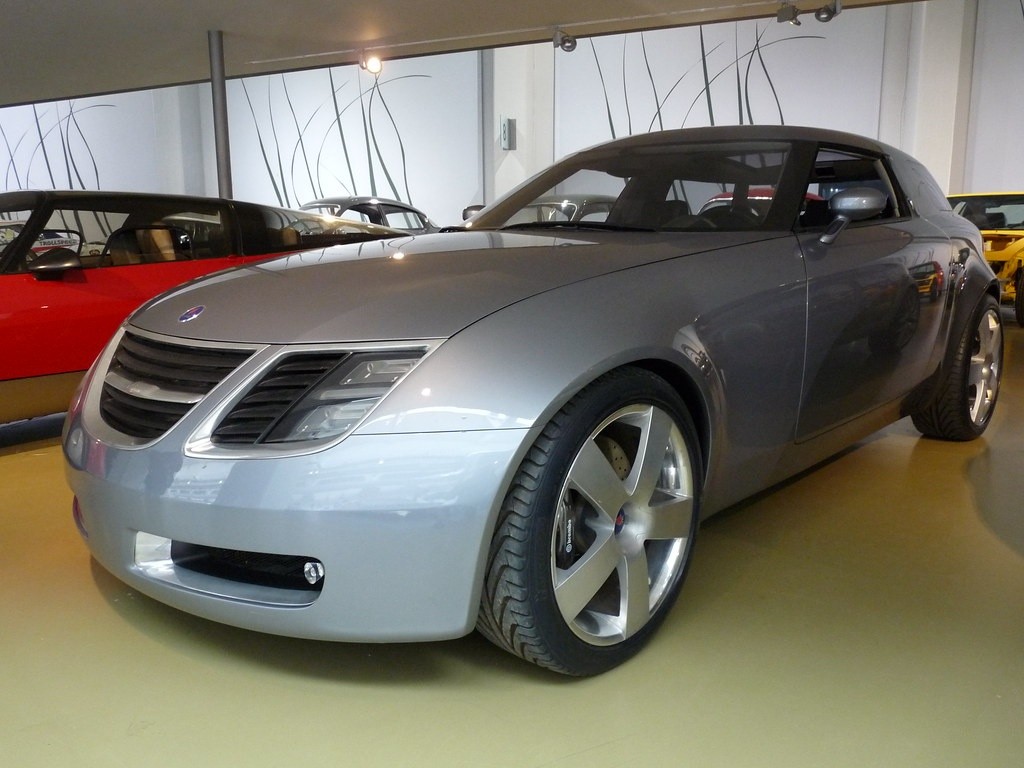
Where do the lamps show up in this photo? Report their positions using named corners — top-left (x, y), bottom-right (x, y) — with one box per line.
top-left (356, 46), bottom-right (382, 74)
top-left (777, 0), bottom-right (802, 26)
top-left (815, 0), bottom-right (842, 23)
top-left (551, 25), bottom-right (577, 52)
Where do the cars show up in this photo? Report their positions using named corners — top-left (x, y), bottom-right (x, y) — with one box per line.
top-left (56, 126), bottom-right (1005, 677)
top-left (0, 191), bottom-right (414, 447)
top-left (947, 192), bottom-right (1024, 328)
top-left (299, 198), bottom-right (445, 236)
top-left (462, 194), bottom-right (621, 225)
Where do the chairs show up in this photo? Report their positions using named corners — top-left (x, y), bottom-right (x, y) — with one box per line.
top-left (800, 200), bottom-right (829, 228)
top-left (657, 200), bottom-right (688, 226)
top-left (99, 225), bottom-right (195, 266)
top-left (40, 229), bottom-right (84, 255)
top-left (986, 212), bottom-right (1006, 228)
